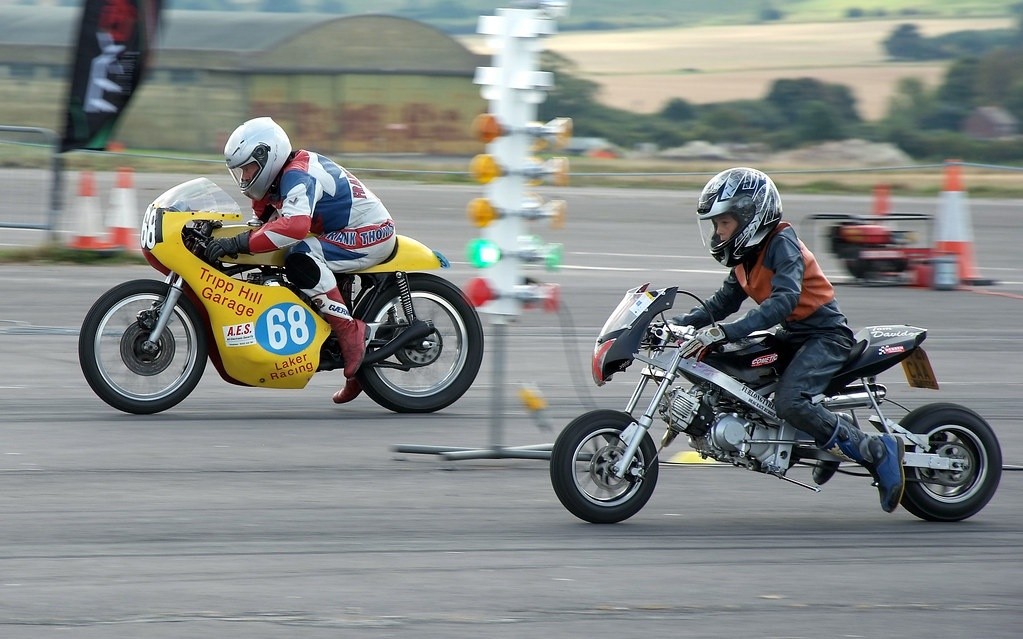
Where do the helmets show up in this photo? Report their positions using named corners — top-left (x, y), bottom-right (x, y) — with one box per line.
top-left (696, 166), bottom-right (784, 267)
top-left (224, 115), bottom-right (293, 202)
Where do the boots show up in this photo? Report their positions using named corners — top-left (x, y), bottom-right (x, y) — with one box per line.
top-left (811, 412), bottom-right (854, 487)
top-left (814, 414), bottom-right (906, 513)
top-left (312, 287), bottom-right (372, 378)
top-left (333, 375), bottom-right (364, 404)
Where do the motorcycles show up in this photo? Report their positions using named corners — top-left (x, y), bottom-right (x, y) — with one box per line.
top-left (549, 281), bottom-right (1003, 525)
top-left (78, 177), bottom-right (485, 415)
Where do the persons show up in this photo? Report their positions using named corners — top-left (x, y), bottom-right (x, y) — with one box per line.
top-left (204, 115), bottom-right (396, 405)
top-left (673, 167), bottom-right (905, 514)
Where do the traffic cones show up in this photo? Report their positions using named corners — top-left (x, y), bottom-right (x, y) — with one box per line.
top-left (99, 163), bottom-right (143, 254)
top-left (913, 158), bottom-right (992, 290)
top-left (63, 167), bottom-right (114, 253)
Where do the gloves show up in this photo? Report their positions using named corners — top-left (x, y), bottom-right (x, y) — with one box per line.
top-left (204, 229), bottom-right (253, 265)
top-left (678, 324), bottom-right (726, 363)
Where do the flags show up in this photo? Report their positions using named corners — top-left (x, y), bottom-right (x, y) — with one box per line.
top-left (57, 0), bottom-right (162, 152)
